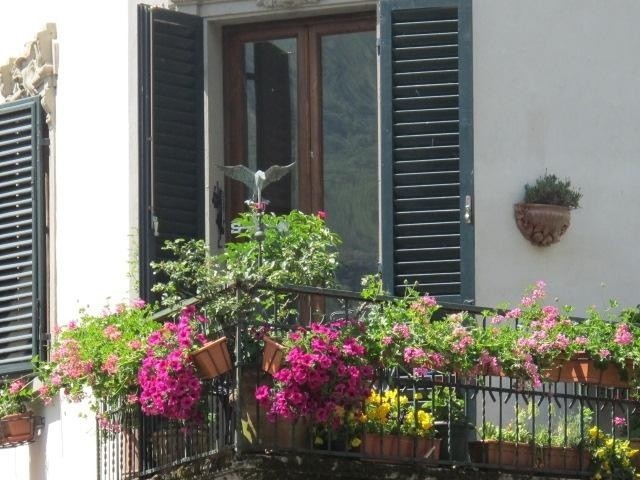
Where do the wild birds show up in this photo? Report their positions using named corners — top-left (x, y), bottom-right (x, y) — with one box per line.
top-left (212, 161), bottom-right (297, 207)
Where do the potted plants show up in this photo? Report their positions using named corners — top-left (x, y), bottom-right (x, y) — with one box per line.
top-left (513, 164), bottom-right (583, 246)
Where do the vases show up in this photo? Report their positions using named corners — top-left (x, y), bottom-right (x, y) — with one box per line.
top-left (0, 416), bottom-right (37, 447)
top-left (359, 418), bottom-right (445, 464)
top-left (535, 350), bottom-right (634, 389)
top-left (190, 334), bottom-right (235, 378)
top-left (467, 438), bottom-right (597, 472)
top-left (260, 332), bottom-right (293, 377)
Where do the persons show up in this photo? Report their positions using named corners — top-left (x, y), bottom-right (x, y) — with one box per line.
top-left (11, 55), bottom-right (30, 100)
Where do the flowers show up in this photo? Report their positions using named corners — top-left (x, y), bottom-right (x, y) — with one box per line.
top-left (349, 385), bottom-right (439, 456)
top-left (254, 317), bottom-right (379, 430)
top-left (0, 373), bottom-right (43, 413)
top-left (385, 279), bottom-right (640, 480)
top-left (128, 304), bottom-right (212, 436)
